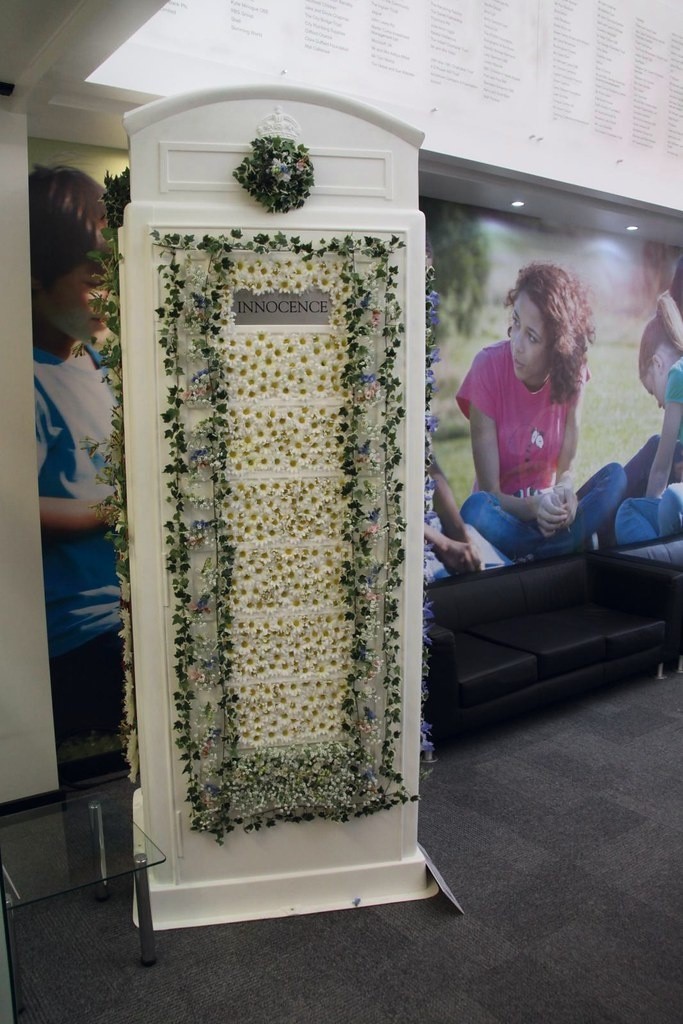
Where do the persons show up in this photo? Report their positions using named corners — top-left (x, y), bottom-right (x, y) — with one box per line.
top-left (422, 452), bottom-right (515, 581)
top-left (28, 162), bottom-right (129, 734)
top-left (613, 254), bottom-right (683, 545)
top-left (455, 261), bottom-right (629, 562)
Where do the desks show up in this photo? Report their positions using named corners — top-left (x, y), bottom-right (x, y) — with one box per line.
top-left (0, 789), bottom-right (167, 1018)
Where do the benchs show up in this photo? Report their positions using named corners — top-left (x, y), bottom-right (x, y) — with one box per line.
top-left (422, 549), bottom-right (683, 765)
top-left (588, 531), bottom-right (683, 572)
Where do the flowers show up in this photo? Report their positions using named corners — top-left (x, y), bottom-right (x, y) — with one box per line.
top-left (92, 136), bottom-right (442, 838)
